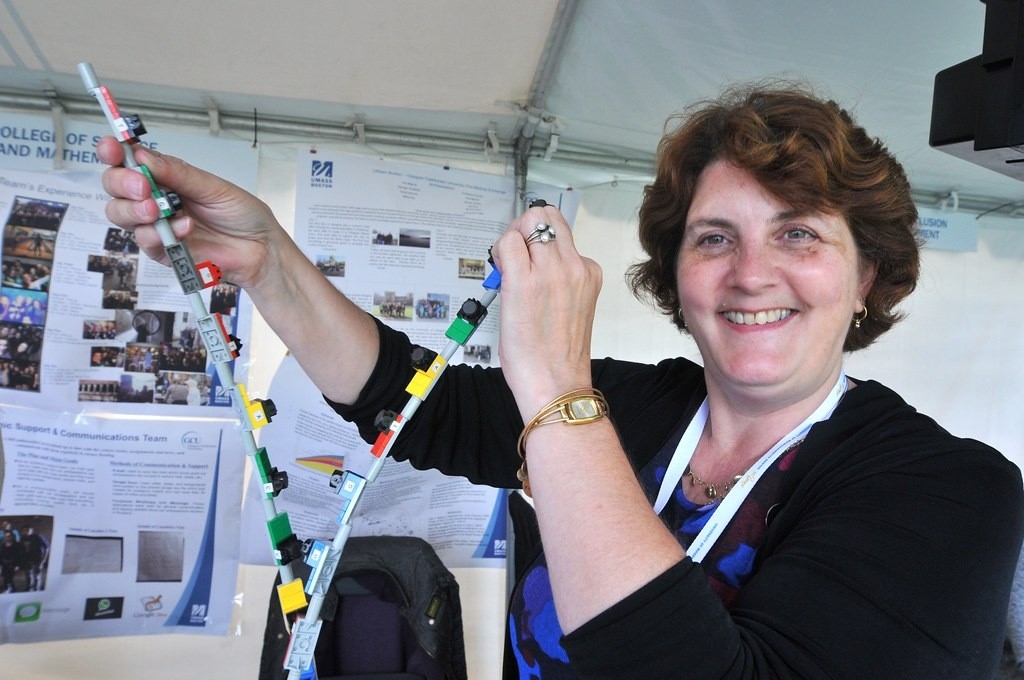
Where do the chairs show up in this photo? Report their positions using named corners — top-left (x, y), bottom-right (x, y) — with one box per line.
top-left (258, 536), bottom-right (468, 680)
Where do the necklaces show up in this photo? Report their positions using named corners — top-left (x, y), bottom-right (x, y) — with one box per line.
top-left (682, 463), bottom-right (751, 506)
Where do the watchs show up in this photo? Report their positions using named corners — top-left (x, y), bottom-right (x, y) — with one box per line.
top-left (523, 395), bottom-right (607, 453)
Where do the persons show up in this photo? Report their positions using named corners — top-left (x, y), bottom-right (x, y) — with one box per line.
top-left (96, 79), bottom-right (1024, 680)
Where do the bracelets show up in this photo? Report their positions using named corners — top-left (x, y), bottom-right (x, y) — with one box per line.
top-left (517, 387), bottom-right (611, 459)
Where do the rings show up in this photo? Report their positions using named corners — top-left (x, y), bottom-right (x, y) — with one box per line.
top-left (526, 223), bottom-right (555, 247)
